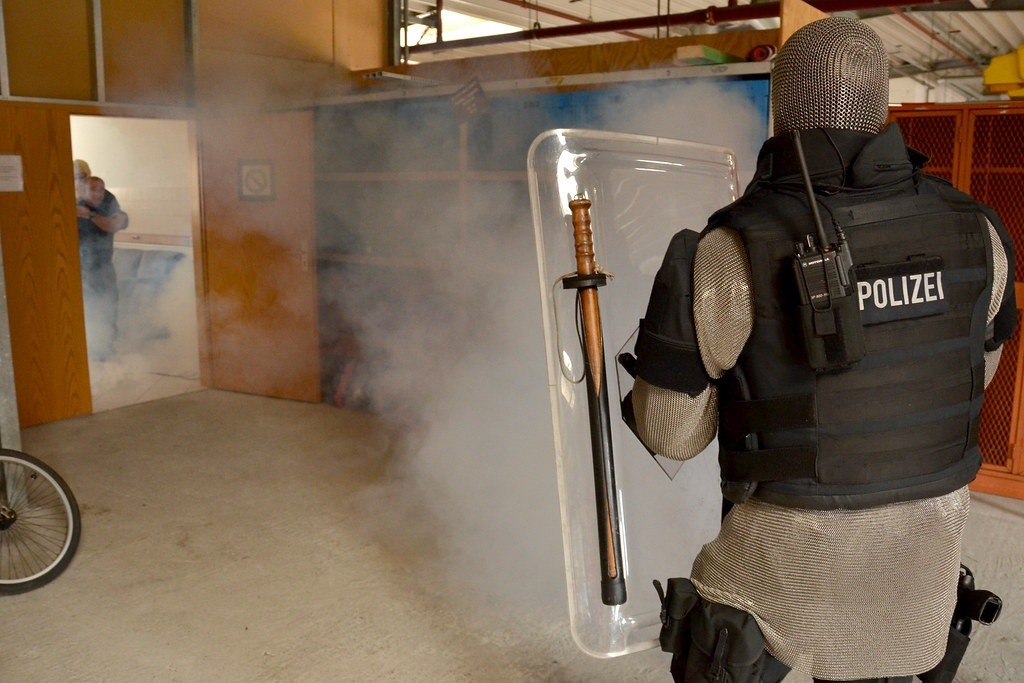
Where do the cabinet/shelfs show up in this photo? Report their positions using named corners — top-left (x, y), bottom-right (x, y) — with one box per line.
top-left (882, 96), bottom-right (1023, 505)
top-left (267, 0), bottom-right (830, 273)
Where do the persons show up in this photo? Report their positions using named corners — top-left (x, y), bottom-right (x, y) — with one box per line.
top-left (74, 160), bottom-right (120, 210)
top-left (76, 177), bottom-right (129, 361)
top-left (616, 19), bottom-right (1020, 682)
